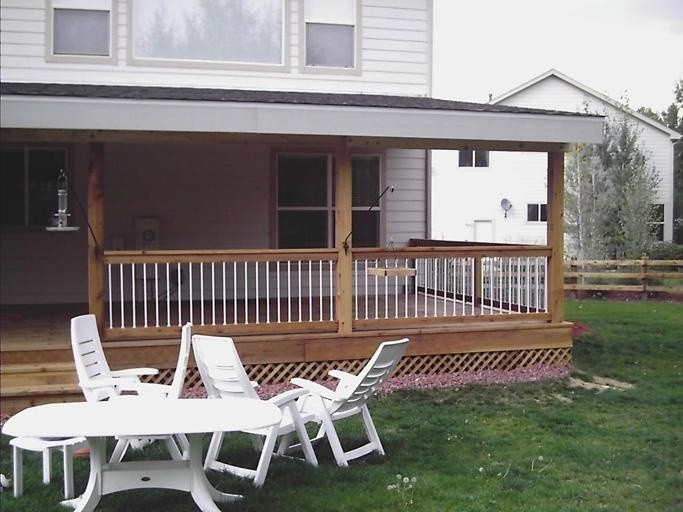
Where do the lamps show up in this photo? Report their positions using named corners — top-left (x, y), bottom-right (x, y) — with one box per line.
top-left (44, 167), bottom-right (103, 256)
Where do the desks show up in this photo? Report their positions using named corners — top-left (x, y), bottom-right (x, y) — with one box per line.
top-left (0, 397), bottom-right (281, 512)
